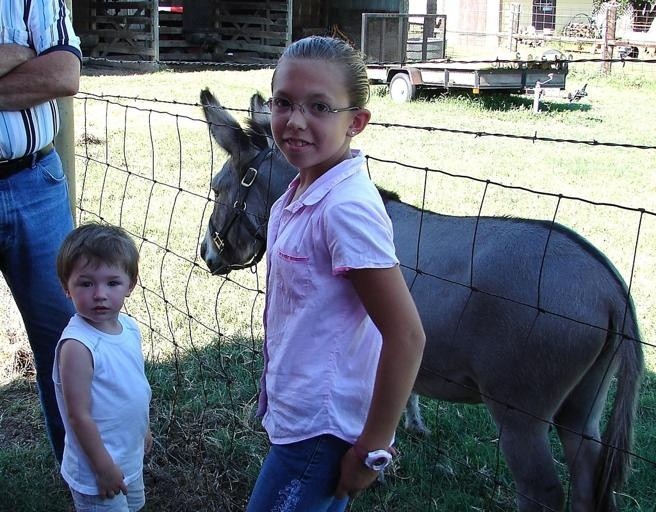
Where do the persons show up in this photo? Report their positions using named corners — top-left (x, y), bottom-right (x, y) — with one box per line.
top-left (43, 215), bottom-right (160, 512)
top-left (241, 36), bottom-right (432, 512)
top-left (0, 0), bottom-right (91, 475)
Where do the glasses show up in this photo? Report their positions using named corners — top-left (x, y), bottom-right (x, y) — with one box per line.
top-left (264, 98), bottom-right (360, 121)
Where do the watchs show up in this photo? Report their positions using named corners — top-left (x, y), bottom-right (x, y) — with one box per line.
top-left (351, 440), bottom-right (399, 470)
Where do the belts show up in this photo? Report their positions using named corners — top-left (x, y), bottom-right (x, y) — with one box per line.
top-left (0, 142), bottom-right (52, 178)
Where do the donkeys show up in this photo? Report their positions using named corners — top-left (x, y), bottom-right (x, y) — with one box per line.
top-left (199, 86), bottom-right (646, 512)
